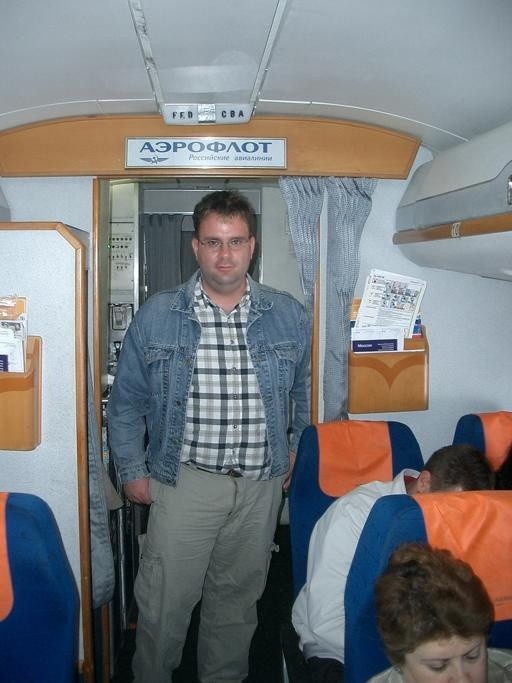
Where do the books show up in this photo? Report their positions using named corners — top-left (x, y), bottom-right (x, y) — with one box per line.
top-left (0, 316), bottom-right (27, 374)
top-left (350, 310), bottom-right (422, 355)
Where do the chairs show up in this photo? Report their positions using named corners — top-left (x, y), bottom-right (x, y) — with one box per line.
top-left (0, 491), bottom-right (79, 683)
top-left (278, 421), bottom-right (426, 683)
top-left (344, 490), bottom-right (512, 682)
top-left (455, 411), bottom-right (511, 490)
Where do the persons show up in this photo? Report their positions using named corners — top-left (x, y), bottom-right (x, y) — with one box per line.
top-left (104, 188), bottom-right (313, 682)
top-left (364, 538), bottom-right (512, 683)
top-left (291, 443), bottom-right (497, 683)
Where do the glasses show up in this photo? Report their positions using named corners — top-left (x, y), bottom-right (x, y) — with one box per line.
top-left (196, 237), bottom-right (251, 250)
